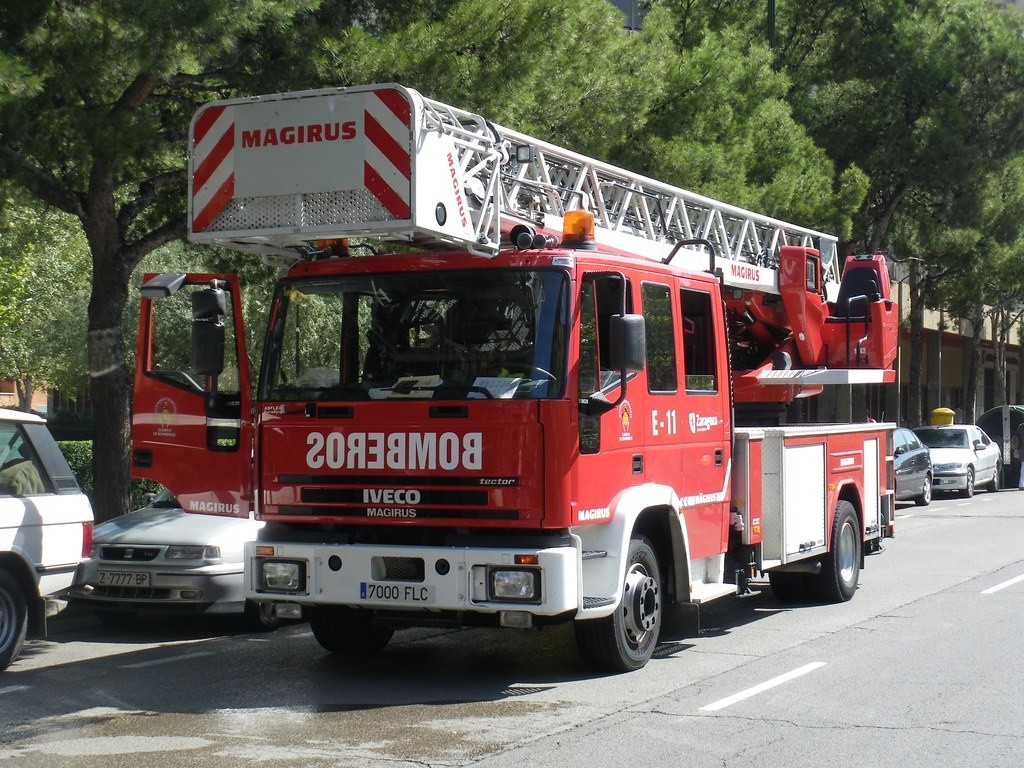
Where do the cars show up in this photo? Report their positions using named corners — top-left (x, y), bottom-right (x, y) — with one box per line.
top-left (61, 488), bottom-right (278, 631)
top-left (892, 427), bottom-right (934, 505)
top-left (912, 424), bottom-right (1003, 497)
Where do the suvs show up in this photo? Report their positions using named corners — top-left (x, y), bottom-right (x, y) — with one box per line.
top-left (0, 408), bottom-right (94, 672)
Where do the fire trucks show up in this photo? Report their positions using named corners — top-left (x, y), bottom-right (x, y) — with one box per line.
top-left (127, 83), bottom-right (899, 671)
